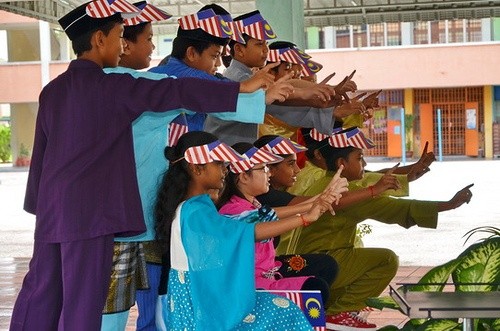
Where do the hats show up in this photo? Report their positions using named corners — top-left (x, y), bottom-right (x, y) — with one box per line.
top-left (168, 140), bottom-right (247, 165)
top-left (233, 9), bottom-right (278, 41)
top-left (123, 0), bottom-right (173, 27)
top-left (302, 127), bottom-right (346, 142)
top-left (58, 0), bottom-right (142, 40)
top-left (176, 8), bottom-right (234, 39)
top-left (226, 146), bottom-right (285, 174)
top-left (267, 46), bottom-right (324, 80)
top-left (259, 136), bottom-right (309, 155)
top-left (317, 127), bottom-right (376, 151)
top-left (220, 15), bottom-right (247, 45)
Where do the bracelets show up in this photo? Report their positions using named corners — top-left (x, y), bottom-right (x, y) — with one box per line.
top-left (369, 186), bottom-right (376, 200)
top-left (296, 214), bottom-right (307, 226)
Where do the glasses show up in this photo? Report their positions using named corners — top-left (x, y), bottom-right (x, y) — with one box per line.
top-left (249, 166), bottom-right (270, 173)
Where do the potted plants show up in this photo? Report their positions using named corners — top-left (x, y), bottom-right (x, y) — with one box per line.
top-left (405, 114), bottom-right (417, 158)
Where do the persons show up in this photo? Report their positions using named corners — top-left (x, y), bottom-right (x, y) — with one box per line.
top-left (151, 129), bottom-right (335, 331)
top-left (9, 0), bottom-right (474, 331)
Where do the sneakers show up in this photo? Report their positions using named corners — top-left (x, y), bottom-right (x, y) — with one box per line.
top-left (325, 312), bottom-right (377, 331)
